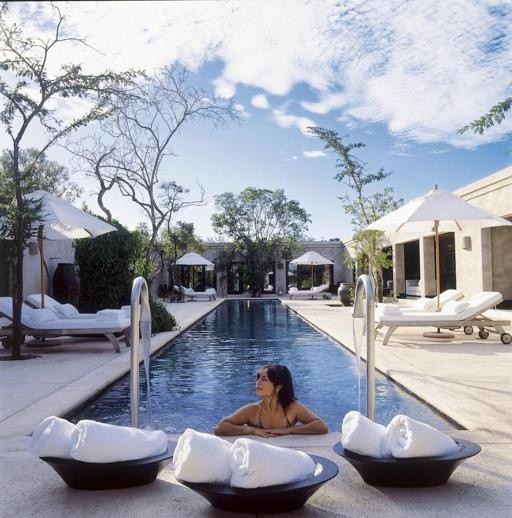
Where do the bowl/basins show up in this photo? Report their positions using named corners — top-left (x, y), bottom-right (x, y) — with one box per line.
top-left (170, 453), bottom-right (340, 511)
top-left (332, 438), bottom-right (481, 488)
top-left (38, 440), bottom-right (178, 490)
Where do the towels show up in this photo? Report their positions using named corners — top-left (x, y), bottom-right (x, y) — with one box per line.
top-left (173, 428), bottom-right (234, 485)
top-left (380, 303), bottom-right (402, 317)
top-left (122, 304), bottom-right (142, 317)
top-left (342, 411), bottom-right (391, 459)
top-left (383, 415), bottom-right (458, 460)
top-left (97, 309), bottom-right (125, 320)
top-left (229, 437), bottom-right (315, 490)
top-left (70, 419), bottom-right (168, 462)
top-left (30, 416), bottom-right (74, 461)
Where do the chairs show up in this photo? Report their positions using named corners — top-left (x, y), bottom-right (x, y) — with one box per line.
top-left (0, 297), bottom-right (131, 354)
top-left (174, 285), bottom-right (217, 301)
top-left (362, 288), bottom-right (464, 335)
top-left (374, 291), bottom-right (512, 345)
top-left (26, 294), bottom-right (144, 338)
top-left (289, 284), bottom-right (329, 299)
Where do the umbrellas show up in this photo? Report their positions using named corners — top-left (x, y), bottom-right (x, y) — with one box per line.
top-left (358, 183), bottom-right (512, 331)
top-left (169, 251), bottom-right (215, 300)
top-left (289, 249), bottom-right (335, 298)
top-left (0, 189), bottom-right (120, 341)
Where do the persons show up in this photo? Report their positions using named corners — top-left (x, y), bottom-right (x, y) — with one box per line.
top-left (213, 364), bottom-right (328, 438)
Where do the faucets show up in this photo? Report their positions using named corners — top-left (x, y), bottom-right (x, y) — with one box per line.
top-left (130, 277), bottom-right (151, 428)
top-left (353, 274), bottom-right (376, 421)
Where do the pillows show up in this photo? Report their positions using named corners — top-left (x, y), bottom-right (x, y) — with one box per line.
top-left (27, 308), bottom-right (59, 323)
top-left (414, 297), bottom-right (434, 308)
top-left (441, 300), bottom-right (470, 314)
top-left (55, 303), bottom-right (78, 316)
